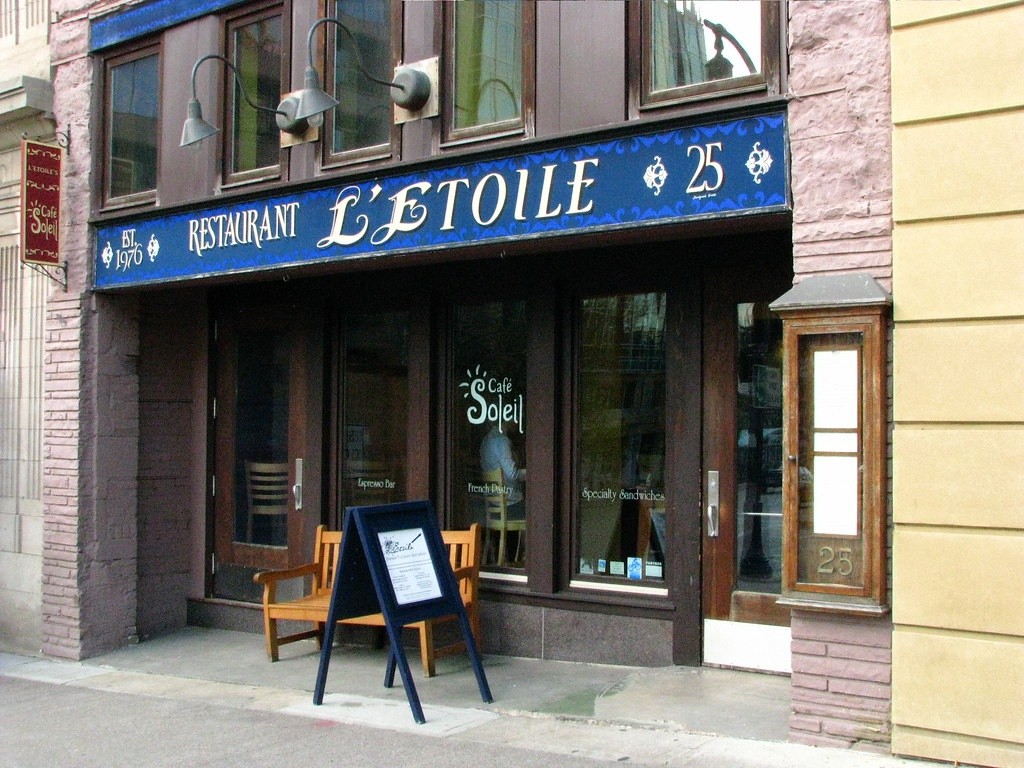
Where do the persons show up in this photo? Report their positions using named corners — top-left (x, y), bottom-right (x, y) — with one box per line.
top-left (479, 400), bottom-right (526, 522)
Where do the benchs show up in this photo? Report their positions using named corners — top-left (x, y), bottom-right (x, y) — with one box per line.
top-left (253, 522), bottom-right (484, 680)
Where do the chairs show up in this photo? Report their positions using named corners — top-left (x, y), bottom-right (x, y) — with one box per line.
top-left (476, 466), bottom-right (528, 569)
top-left (240, 459), bottom-right (292, 544)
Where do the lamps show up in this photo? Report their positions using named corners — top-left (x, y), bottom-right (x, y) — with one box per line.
top-left (294, 18), bottom-right (431, 130)
top-left (180, 54), bottom-right (310, 152)
